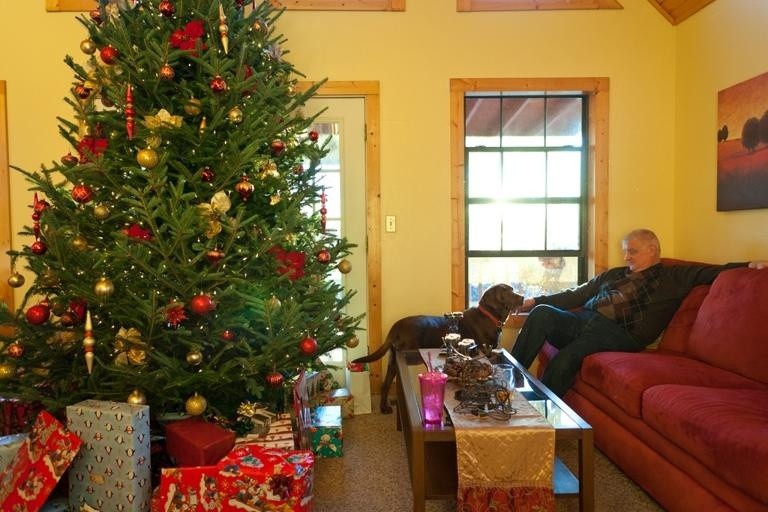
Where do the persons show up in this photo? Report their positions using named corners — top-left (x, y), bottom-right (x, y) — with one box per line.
top-left (507, 227), bottom-right (768, 396)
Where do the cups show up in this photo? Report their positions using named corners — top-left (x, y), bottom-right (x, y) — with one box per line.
top-left (492, 362), bottom-right (516, 405)
top-left (416, 371), bottom-right (449, 425)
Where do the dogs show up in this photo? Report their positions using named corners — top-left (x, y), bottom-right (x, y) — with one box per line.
top-left (351, 284), bottom-right (525, 415)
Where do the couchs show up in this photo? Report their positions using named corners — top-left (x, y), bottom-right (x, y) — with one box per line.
top-left (538, 258), bottom-right (767, 512)
